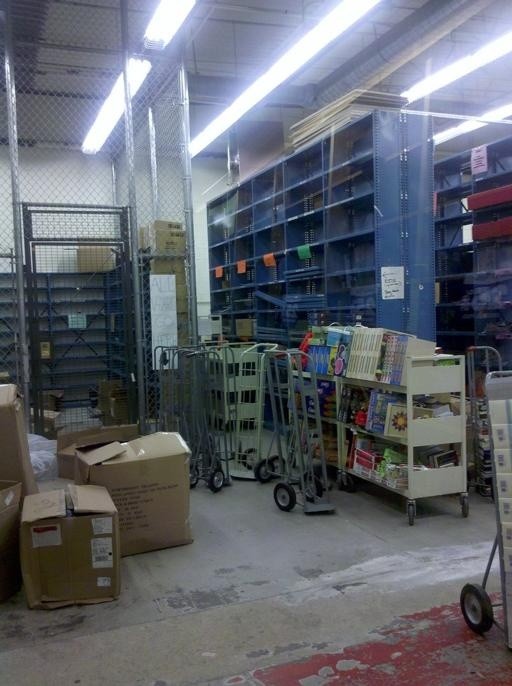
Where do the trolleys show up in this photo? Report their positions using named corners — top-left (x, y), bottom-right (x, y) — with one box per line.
top-left (458, 368), bottom-right (512, 652)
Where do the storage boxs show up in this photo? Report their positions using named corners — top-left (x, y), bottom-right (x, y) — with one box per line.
top-left (0, 371), bottom-right (193, 611)
top-left (138, 219), bottom-right (192, 371)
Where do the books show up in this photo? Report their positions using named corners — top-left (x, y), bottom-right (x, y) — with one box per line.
top-left (476, 427), bottom-right (491, 485)
top-left (418, 447), bottom-right (458, 468)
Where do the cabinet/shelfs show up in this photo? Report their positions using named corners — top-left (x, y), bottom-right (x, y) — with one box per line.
top-left (207, 107), bottom-right (512, 525)
top-left (0, 245), bottom-right (151, 431)
